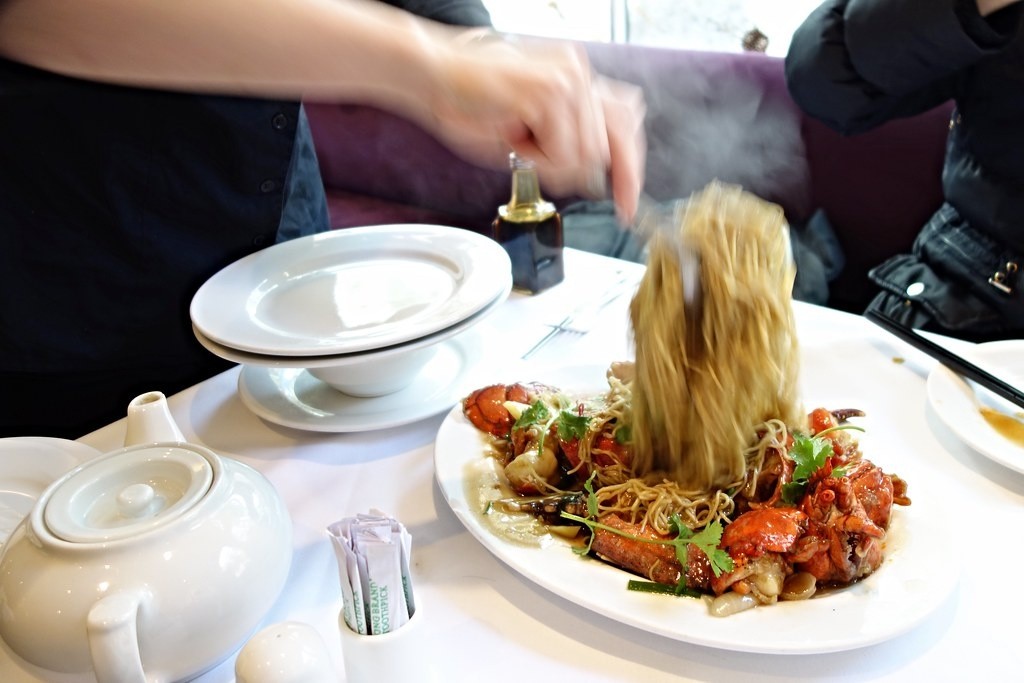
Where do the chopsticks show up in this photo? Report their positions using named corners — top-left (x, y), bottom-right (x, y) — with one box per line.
top-left (866, 307), bottom-right (1024, 408)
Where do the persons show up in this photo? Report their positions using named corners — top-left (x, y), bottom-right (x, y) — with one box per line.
top-left (777, 0), bottom-right (1024, 349)
top-left (0, 2), bottom-right (652, 438)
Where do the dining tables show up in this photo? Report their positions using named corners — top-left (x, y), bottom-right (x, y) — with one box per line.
top-left (0, 244), bottom-right (1024, 683)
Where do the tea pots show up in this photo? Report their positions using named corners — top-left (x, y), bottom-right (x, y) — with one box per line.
top-left (0, 390), bottom-right (296, 683)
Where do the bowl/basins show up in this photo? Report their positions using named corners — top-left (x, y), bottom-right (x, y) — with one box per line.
top-left (305, 345), bottom-right (443, 398)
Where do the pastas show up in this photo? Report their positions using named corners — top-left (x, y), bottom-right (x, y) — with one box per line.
top-left (530, 179), bottom-right (802, 536)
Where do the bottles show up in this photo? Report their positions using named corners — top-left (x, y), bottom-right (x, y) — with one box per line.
top-left (491, 152), bottom-right (564, 296)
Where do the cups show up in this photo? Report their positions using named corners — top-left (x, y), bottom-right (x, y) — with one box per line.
top-left (338, 601), bottom-right (431, 683)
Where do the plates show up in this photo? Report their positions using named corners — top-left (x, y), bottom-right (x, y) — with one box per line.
top-left (927, 340), bottom-right (1024, 474)
top-left (238, 324), bottom-right (505, 435)
top-left (191, 224), bottom-right (514, 368)
top-left (0, 437), bottom-right (104, 547)
top-left (434, 361), bottom-right (989, 655)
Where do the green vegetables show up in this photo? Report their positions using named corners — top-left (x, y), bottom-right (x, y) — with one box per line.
top-left (559, 469), bottom-right (734, 577)
top-left (512, 398), bottom-right (591, 456)
top-left (779, 426), bottom-right (866, 505)
